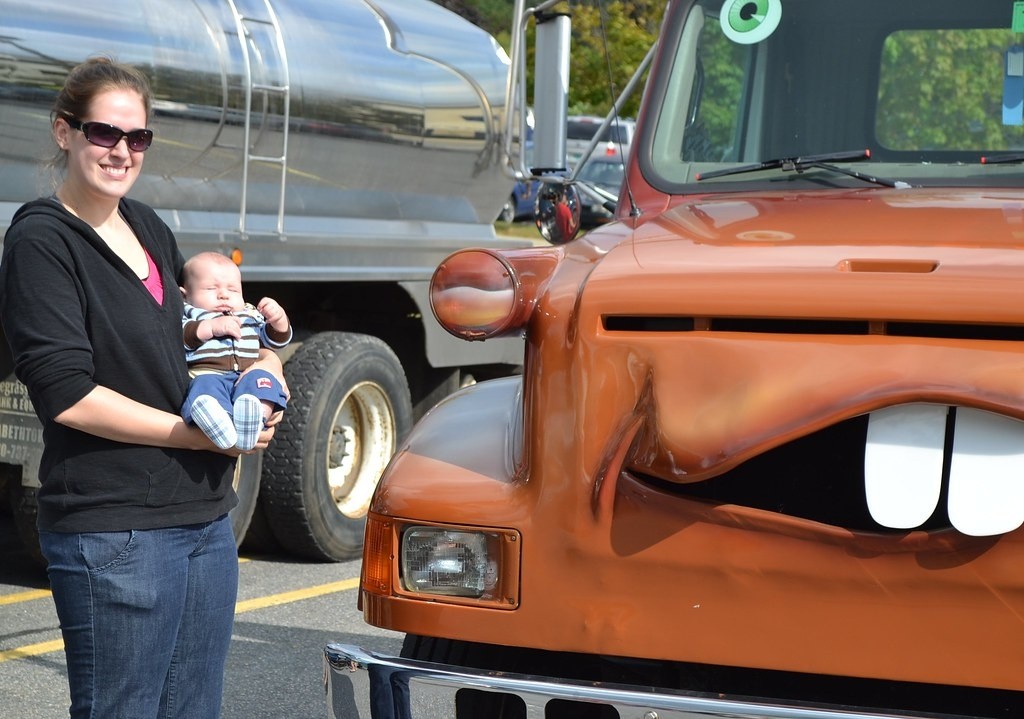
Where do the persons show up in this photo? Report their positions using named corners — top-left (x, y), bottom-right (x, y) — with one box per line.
top-left (178, 251), bottom-right (293, 452)
top-left (537, 184), bottom-right (576, 242)
top-left (0, 58), bottom-right (291, 719)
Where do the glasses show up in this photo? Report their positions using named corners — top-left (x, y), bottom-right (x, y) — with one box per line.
top-left (68, 119), bottom-right (153, 152)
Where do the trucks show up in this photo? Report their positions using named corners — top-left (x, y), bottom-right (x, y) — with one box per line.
top-left (0, 0), bottom-right (551, 562)
top-left (322, 0), bottom-right (1024, 719)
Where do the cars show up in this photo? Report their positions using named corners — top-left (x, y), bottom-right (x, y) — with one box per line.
top-left (499, 115), bottom-right (636, 223)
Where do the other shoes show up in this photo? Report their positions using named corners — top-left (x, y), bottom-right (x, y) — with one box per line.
top-left (233, 393), bottom-right (263, 450)
top-left (190, 394), bottom-right (238, 449)
